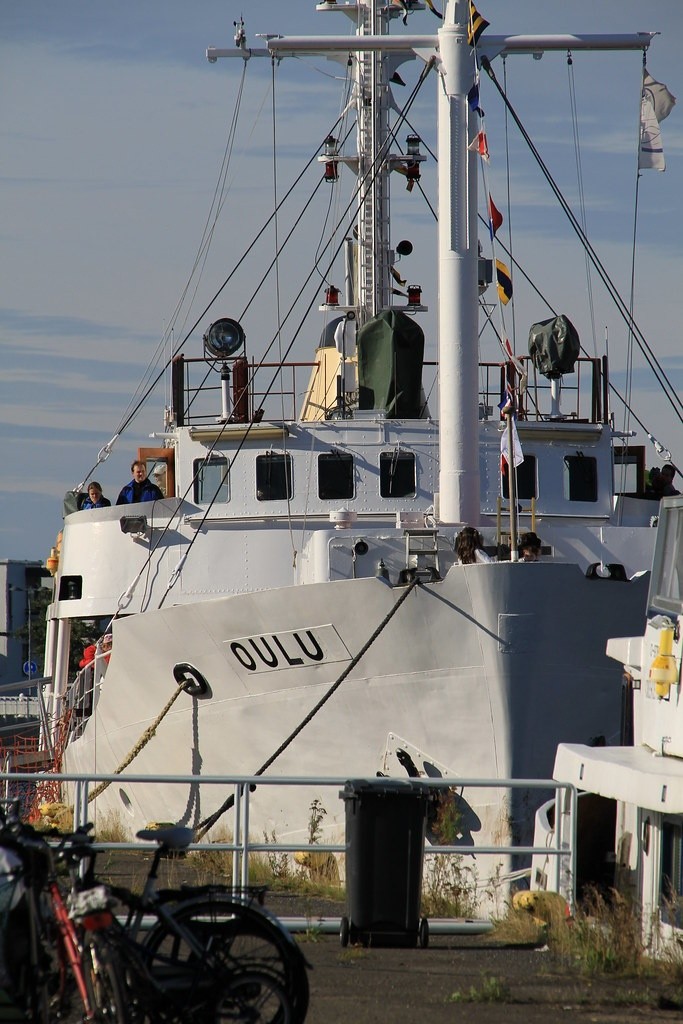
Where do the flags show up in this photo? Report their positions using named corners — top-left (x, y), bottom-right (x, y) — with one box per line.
top-left (497, 382), bottom-right (517, 420)
top-left (501, 417), bottom-right (524, 469)
top-left (468, 84), bottom-right (485, 118)
top-left (638, 68), bottom-right (676, 172)
top-left (468, 131), bottom-right (491, 169)
top-left (501, 325), bottom-right (527, 377)
top-left (494, 259), bottom-right (513, 305)
top-left (468, 0), bottom-right (490, 48)
top-left (487, 190), bottom-right (503, 240)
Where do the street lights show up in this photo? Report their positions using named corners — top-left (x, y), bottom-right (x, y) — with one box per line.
top-left (8, 585), bottom-right (50, 696)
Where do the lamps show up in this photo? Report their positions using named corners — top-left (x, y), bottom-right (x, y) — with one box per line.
top-left (204, 318), bottom-right (246, 358)
top-left (119, 515), bottom-right (148, 534)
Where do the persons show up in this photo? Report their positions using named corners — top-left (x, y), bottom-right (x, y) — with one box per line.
top-left (647, 464), bottom-right (681, 500)
top-left (518, 532), bottom-right (543, 561)
top-left (115, 460), bottom-right (165, 505)
top-left (152, 464), bottom-right (167, 496)
top-left (80, 481), bottom-right (111, 511)
top-left (452, 526), bottom-right (497, 564)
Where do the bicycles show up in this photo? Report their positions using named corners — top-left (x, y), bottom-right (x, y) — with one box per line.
top-left (0, 752), bottom-right (312, 1024)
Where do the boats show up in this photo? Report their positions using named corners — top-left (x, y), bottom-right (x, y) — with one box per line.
top-left (0, 355), bottom-right (683, 917)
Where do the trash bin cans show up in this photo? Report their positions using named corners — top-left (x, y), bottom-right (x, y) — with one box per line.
top-left (336, 780), bottom-right (435, 948)
